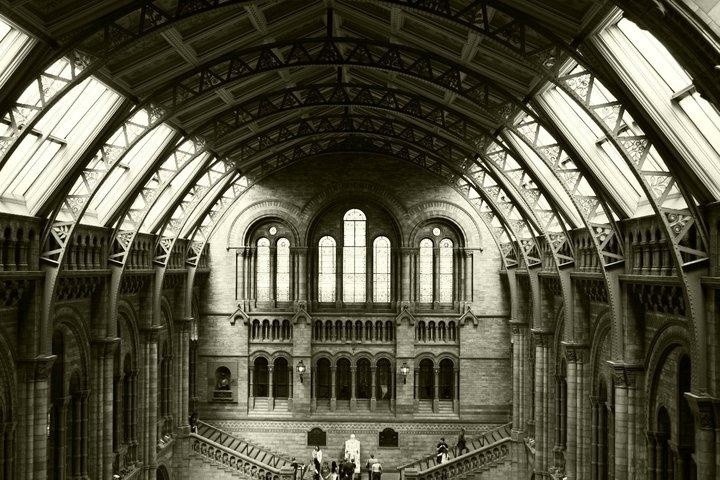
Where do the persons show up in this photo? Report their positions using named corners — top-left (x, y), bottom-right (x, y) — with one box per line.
top-left (345, 434), bottom-right (361, 468)
top-left (289, 445), bottom-right (383, 480)
top-left (436, 437), bottom-right (449, 464)
top-left (457, 427), bottom-right (470, 457)
top-left (437, 449), bottom-right (451, 464)
